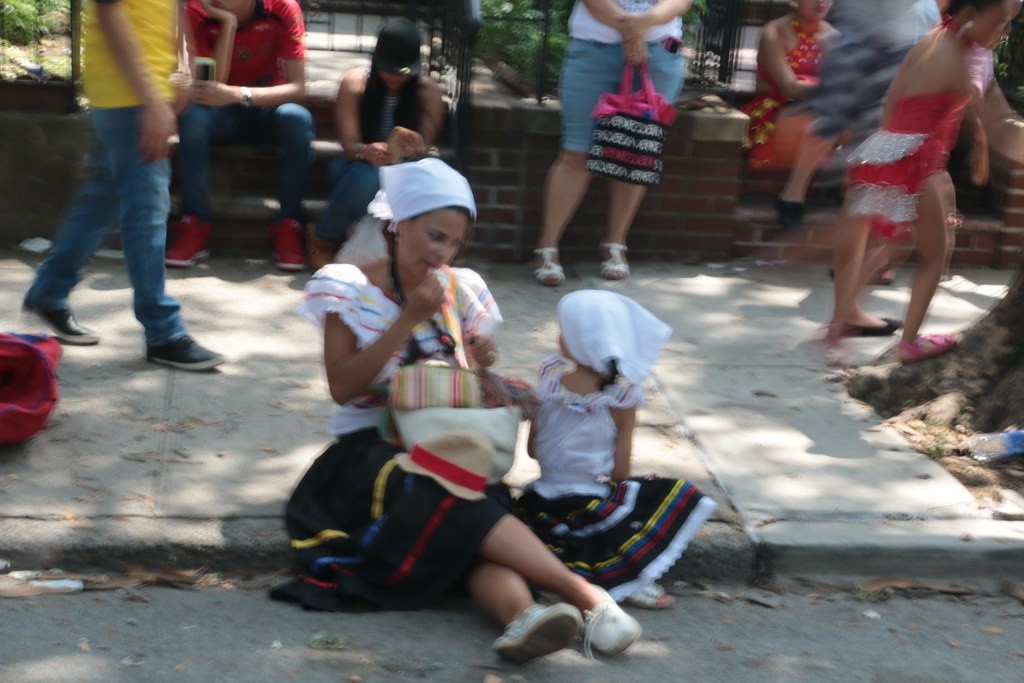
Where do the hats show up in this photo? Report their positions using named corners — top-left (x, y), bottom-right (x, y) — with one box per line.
top-left (375, 16), bottom-right (421, 75)
top-left (394, 427), bottom-right (494, 504)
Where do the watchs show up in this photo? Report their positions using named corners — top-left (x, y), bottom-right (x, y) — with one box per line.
top-left (238, 86), bottom-right (254, 107)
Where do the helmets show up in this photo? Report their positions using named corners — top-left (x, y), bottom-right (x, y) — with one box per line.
top-left (1, 333), bottom-right (64, 447)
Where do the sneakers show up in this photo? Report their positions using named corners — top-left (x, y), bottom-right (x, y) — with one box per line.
top-left (146, 335), bottom-right (225, 370)
top-left (163, 213), bottom-right (212, 268)
top-left (578, 584), bottom-right (642, 661)
top-left (22, 296), bottom-right (100, 345)
top-left (490, 602), bottom-right (583, 664)
top-left (270, 216), bottom-right (308, 271)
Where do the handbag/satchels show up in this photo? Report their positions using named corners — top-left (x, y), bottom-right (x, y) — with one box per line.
top-left (388, 358), bottom-right (526, 482)
top-left (584, 54), bottom-right (676, 188)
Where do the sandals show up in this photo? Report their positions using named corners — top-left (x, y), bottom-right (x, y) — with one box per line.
top-left (532, 246), bottom-right (566, 285)
top-left (597, 237), bottom-right (630, 281)
top-left (624, 582), bottom-right (676, 609)
top-left (896, 334), bottom-right (958, 365)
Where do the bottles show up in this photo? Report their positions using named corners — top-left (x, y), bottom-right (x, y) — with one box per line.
top-left (968, 428), bottom-right (1024, 461)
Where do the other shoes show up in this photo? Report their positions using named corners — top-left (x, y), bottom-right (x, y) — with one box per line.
top-left (775, 191), bottom-right (808, 233)
top-left (829, 314), bottom-right (903, 335)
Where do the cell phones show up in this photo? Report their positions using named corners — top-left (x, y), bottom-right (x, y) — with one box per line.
top-left (385, 125), bottom-right (408, 164)
top-left (665, 36), bottom-right (679, 53)
top-left (194, 57), bottom-right (215, 82)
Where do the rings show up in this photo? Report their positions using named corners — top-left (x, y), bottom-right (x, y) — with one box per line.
top-left (404, 145), bottom-right (412, 151)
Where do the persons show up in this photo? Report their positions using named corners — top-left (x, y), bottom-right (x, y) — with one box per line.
top-left (23, 0), bottom-right (227, 370)
top-left (746, 0), bottom-right (1024, 363)
top-left (306, 21), bottom-right (460, 275)
top-left (309, 162), bottom-right (644, 664)
top-left (519, 292), bottom-right (713, 611)
top-left (164, 0), bottom-right (316, 273)
top-left (529, 0), bottom-right (692, 289)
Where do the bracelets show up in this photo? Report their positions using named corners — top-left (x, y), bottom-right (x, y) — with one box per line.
top-left (354, 143), bottom-right (368, 161)
